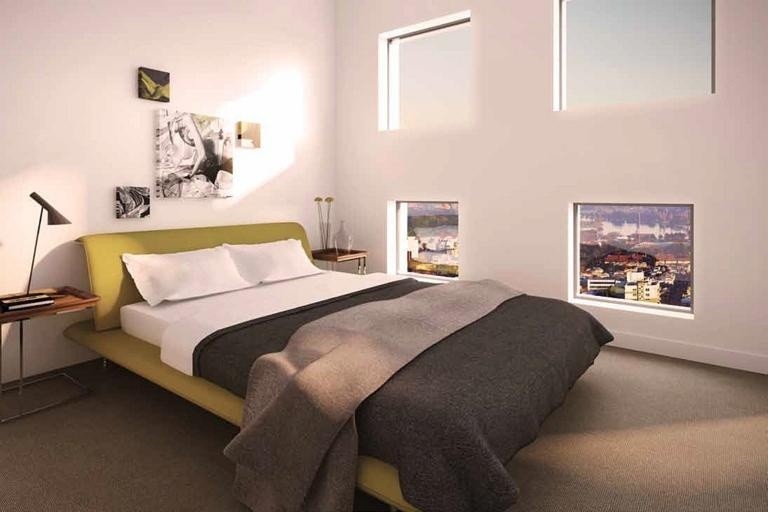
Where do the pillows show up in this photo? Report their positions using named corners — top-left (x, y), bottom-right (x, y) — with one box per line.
top-left (223, 238), bottom-right (325, 286)
top-left (122, 245), bottom-right (254, 307)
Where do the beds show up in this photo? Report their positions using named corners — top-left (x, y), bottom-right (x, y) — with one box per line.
top-left (63, 222), bottom-right (616, 512)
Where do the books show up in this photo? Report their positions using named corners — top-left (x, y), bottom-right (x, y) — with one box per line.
top-left (0, 293), bottom-right (54, 311)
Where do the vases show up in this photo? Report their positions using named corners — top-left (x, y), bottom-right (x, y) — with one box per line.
top-left (319, 222), bottom-right (331, 253)
top-left (335, 220), bottom-right (353, 255)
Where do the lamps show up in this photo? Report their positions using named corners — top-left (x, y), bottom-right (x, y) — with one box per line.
top-left (25, 189), bottom-right (73, 299)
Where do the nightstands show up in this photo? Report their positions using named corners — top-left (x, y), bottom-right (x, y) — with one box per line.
top-left (314, 249), bottom-right (369, 276)
top-left (1, 286), bottom-right (101, 425)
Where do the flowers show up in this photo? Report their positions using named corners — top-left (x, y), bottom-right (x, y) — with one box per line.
top-left (315, 196), bottom-right (333, 250)
